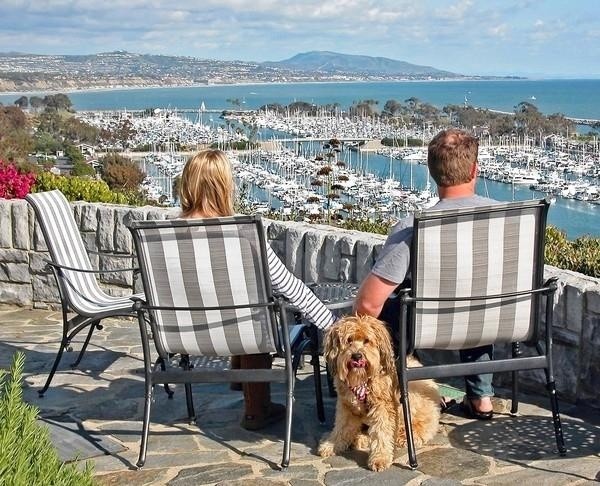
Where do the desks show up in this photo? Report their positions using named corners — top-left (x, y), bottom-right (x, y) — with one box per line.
top-left (277, 281), bottom-right (362, 396)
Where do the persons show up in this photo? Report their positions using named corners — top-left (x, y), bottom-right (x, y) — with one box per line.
top-left (353, 128), bottom-right (507, 419)
top-left (176, 149), bottom-right (341, 430)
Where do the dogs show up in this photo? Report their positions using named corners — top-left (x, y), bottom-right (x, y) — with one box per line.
top-left (314, 310), bottom-right (442, 473)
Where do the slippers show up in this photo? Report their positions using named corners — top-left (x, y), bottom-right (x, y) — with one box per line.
top-left (462, 394), bottom-right (493, 420)
top-left (240, 403), bottom-right (286, 430)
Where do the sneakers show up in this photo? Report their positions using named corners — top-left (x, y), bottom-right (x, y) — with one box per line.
top-left (230, 383), bottom-right (242, 391)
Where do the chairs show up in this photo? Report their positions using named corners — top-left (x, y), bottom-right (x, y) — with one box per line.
top-left (24, 189), bottom-right (174, 402)
top-left (392, 197), bottom-right (566, 467)
top-left (125, 214), bottom-right (328, 468)
